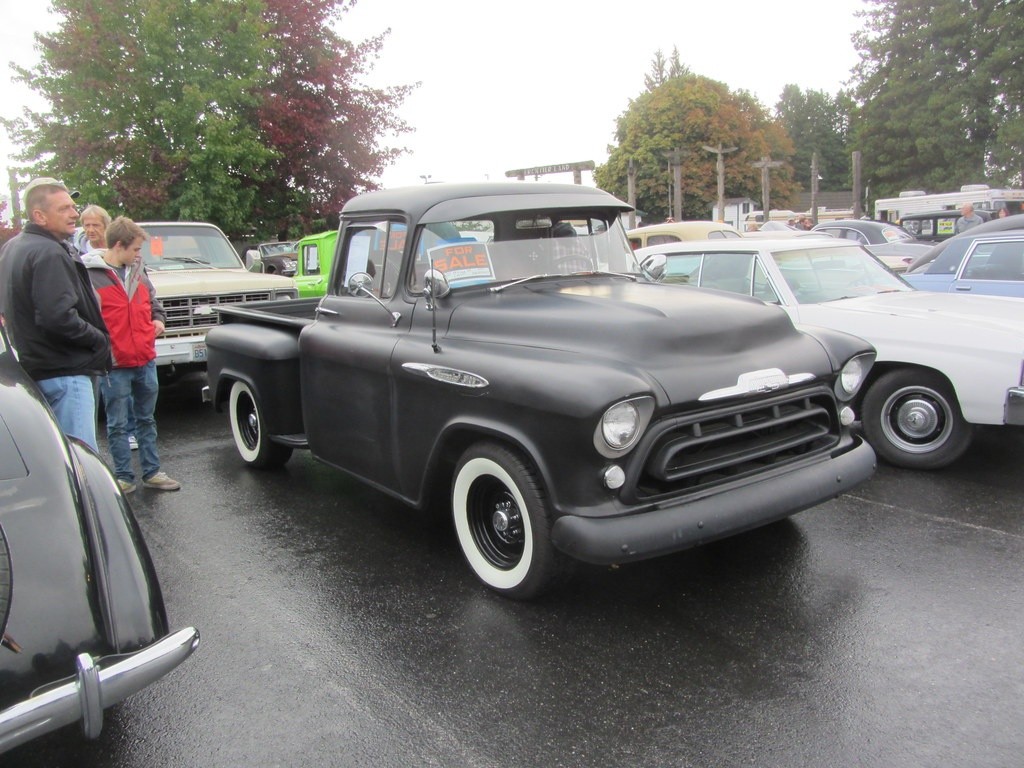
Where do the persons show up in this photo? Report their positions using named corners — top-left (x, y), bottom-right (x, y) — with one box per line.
top-left (746, 222), bottom-right (761, 232)
top-left (80, 216), bottom-right (182, 496)
top-left (787, 214), bottom-right (814, 231)
top-left (73, 204), bottom-right (139, 450)
top-left (859, 211), bottom-right (873, 220)
top-left (996, 207), bottom-right (1009, 219)
top-left (0, 177), bottom-right (113, 454)
top-left (955, 204), bottom-right (984, 235)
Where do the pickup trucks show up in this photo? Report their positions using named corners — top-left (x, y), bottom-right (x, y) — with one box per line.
top-left (202, 182), bottom-right (877, 602)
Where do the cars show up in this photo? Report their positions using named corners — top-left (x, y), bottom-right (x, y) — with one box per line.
top-left (0, 321), bottom-right (200, 755)
top-left (626, 185), bottom-right (1024, 471)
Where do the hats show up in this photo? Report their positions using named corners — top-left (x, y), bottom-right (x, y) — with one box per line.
top-left (23, 177), bottom-right (80, 211)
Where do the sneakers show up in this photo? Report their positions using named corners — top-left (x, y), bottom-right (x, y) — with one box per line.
top-left (143, 471), bottom-right (180, 490)
top-left (128, 436), bottom-right (138, 450)
top-left (117, 479), bottom-right (136, 495)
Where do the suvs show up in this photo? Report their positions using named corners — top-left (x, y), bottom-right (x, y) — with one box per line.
top-left (69, 222), bottom-right (338, 422)
